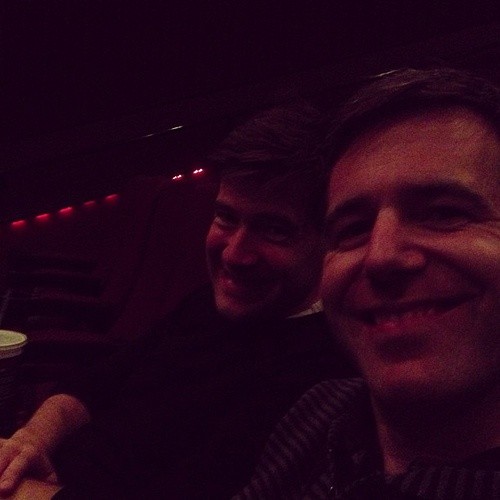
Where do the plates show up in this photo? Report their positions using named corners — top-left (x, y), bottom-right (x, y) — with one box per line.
top-left (1, 329), bottom-right (28, 360)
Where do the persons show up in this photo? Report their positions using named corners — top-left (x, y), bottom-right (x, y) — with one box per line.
top-left (220, 66), bottom-right (500, 500)
top-left (0, 103), bottom-right (362, 500)
